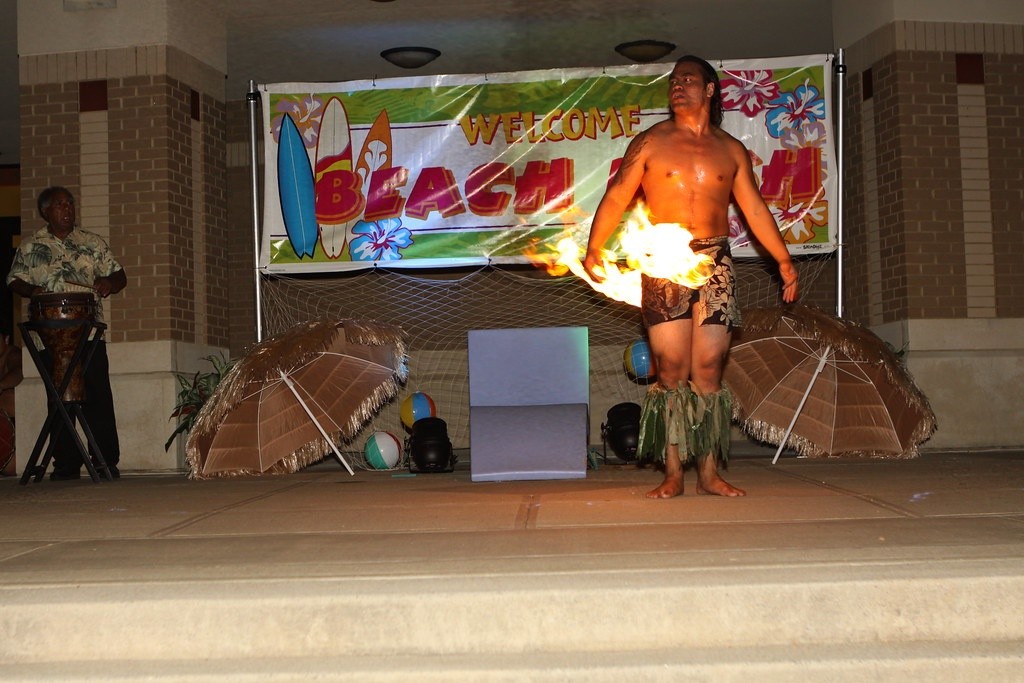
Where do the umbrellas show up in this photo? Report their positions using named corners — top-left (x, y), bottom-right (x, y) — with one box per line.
top-left (720, 296), bottom-right (938, 466)
top-left (184, 314), bottom-right (409, 477)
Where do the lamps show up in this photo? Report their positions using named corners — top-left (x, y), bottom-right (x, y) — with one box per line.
top-left (380, 46), bottom-right (441, 68)
top-left (615, 39), bottom-right (677, 62)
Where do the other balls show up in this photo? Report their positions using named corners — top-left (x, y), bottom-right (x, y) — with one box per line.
top-left (624, 337), bottom-right (658, 379)
top-left (399, 391), bottom-right (436, 430)
top-left (363, 430), bottom-right (403, 469)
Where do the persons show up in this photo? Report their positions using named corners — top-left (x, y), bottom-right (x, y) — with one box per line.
top-left (584, 52), bottom-right (799, 501)
top-left (3, 186), bottom-right (128, 479)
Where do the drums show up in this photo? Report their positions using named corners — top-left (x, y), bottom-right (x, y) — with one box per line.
top-left (28, 291), bottom-right (98, 414)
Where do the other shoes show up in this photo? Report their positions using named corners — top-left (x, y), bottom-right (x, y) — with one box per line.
top-left (93, 462), bottom-right (120, 479)
top-left (49, 465), bottom-right (81, 480)
